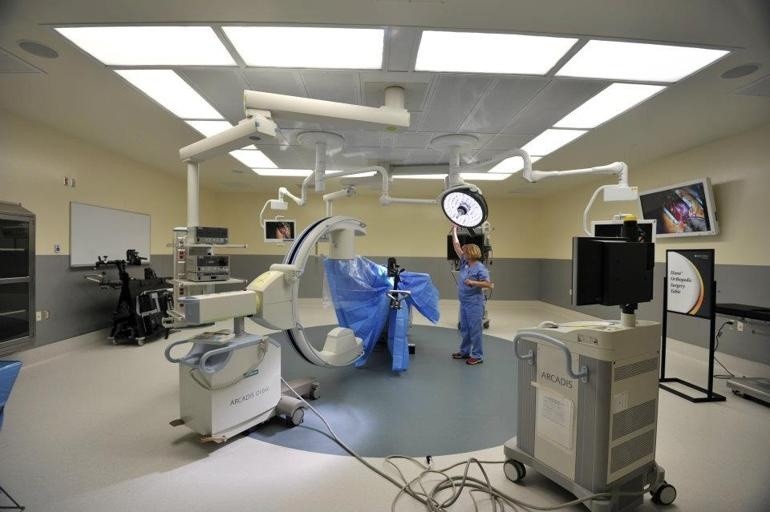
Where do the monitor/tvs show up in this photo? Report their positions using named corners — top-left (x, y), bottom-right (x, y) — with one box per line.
top-left (637, 177), bottom-right (722, 238)
top-left (263, 219), bottom-right (297, 241)
top-left (570, 236), bottom-right (655, 308)
top-left (592, 219), bottom-right (658, 244)
top-left (446, 234), bottom-right (485, 262)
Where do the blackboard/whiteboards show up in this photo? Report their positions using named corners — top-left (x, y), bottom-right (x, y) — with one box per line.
top-left (70, 201), bottom-right (151, 268)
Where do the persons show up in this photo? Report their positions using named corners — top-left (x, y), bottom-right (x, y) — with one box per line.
top-left (450, 222), bottom-right (493, 365)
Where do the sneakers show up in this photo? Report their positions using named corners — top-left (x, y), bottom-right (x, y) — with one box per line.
top-left (453, 352), bottom-right (483, 364)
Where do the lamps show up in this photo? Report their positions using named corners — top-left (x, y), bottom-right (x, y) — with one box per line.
top-left (442, 185), bottom-right (488, 229)
